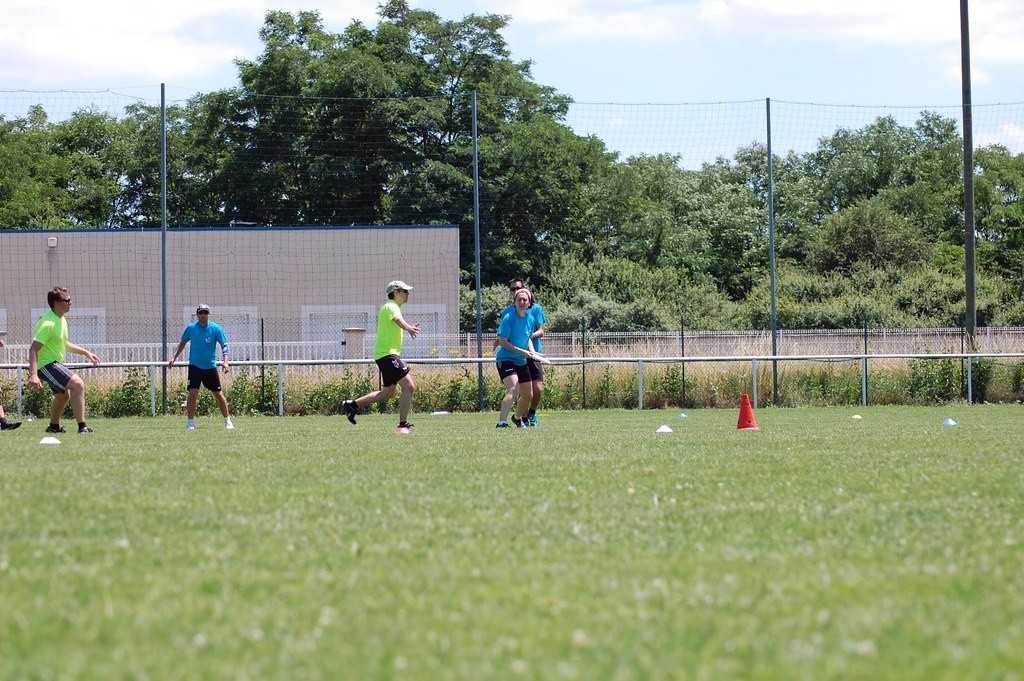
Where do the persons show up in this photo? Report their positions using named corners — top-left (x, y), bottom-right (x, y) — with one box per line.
top-left (0, 340), bottom-right (22, 430)
top-left (343, 281), bottom-right (421, 429)
top-left (493, 278), bottom-right (546, 429)
top-left (168, 303), bottom-right (235, 429)
top-left (29, 286), bottom-right (100, 433)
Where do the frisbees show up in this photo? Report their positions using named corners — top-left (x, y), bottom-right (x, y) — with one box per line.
top-left (529, 352), bottom-right (552, 365)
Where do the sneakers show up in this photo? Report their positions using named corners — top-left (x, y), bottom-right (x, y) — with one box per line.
top-left (225, 419), bottom-right (234, 429)
top-left (187, 420), bottom-right (194, 430)
top-left (46, 426), bottom-right (66, 433)
top-left (343, 400), bottom-right (359, 425)
top-left (79, 427), bottom-right (93, 433)
top-left (513, 414), bottom-right (527, 428)
top-left (523, 409), bottom-right (538, 426)
top-left (397, 423), bottom-right (414, 433)
top-left (1, 421), bottom-right (22, 430)
top-left (496, 423), bottom-right (512, 428)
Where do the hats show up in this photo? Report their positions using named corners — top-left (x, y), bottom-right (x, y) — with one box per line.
top-left (197, 304), bottom-right (209, 311)
top-left (387, 280), bottom-right (413, 293)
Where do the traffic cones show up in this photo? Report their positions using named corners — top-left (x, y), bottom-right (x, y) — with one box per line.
top-left (736, 394), bottom-right (759, 432)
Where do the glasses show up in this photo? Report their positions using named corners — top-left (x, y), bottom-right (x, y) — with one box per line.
top-left (511, 287), bottom-right (521, 291)
top-left (199, 311), bottom-right (209, 315)
top-left (401, 290), bottom-right (408, 293)
top-left (60, 299), bottom-right (71, 302)
